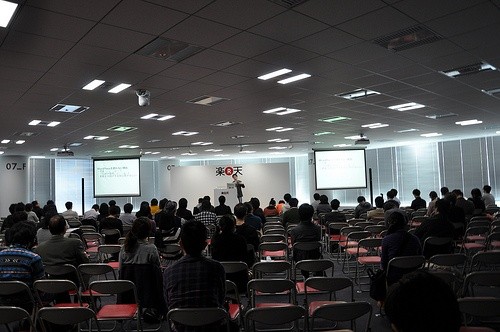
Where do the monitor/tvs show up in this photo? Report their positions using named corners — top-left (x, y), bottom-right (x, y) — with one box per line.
top-left (227, 183), bottom-right (234, 189)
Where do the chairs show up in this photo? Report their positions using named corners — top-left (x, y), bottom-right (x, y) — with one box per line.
top-left (0, 206), bottom-right (500, 332)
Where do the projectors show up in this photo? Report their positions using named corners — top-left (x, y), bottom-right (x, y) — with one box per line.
top-left (57, 152), bottom-right (74, 157)
top-left (355, 139), bottom-right (370, 144)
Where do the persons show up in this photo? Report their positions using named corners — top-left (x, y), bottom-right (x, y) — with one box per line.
top-left (0, 174), bottom-right (495, 332)
